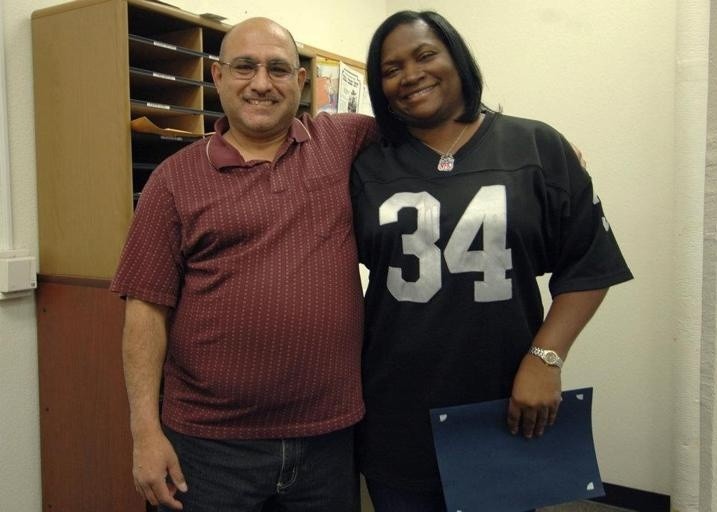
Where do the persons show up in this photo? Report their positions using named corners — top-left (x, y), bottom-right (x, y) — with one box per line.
top-left (104, 16), bottom-right (380, 512)
top-left (348, 8), bottom-right (634, 512)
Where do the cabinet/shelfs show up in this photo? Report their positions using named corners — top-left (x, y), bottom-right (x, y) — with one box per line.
top-left (28, 268), bottom-right (162, 510)
top-left (24, 1), bottom-right (378, 290)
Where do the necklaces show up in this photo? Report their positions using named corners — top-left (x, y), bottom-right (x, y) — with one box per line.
top-left (418, 121), bottom-right (468, 173)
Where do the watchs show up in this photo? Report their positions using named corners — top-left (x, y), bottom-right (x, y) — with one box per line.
top-left (528, 344), bottom-right (564, 370)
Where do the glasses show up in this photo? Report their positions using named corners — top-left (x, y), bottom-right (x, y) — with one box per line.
top-left (218, 57), bottom-right (299, 81)
